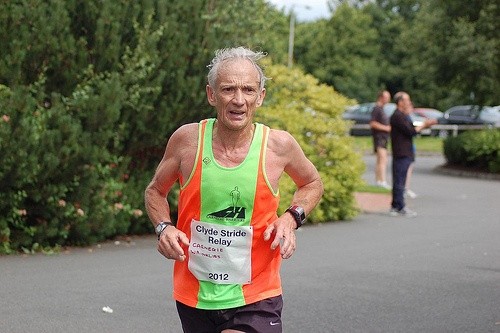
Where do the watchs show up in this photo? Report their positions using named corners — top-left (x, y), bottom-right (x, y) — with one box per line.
top-left (283, 205), bottom-right (308, 229)
top-left (155, 221), bottom-right (173, 240)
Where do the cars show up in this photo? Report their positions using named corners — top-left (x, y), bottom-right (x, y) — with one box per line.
top-left (340, 102), bottom-right (500, 139)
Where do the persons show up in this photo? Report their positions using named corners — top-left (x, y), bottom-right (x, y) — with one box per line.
top-left (145, 46), bottom-right (323, 333)
top-left (368, 90), bottom-right (394, 192)
top-left (389, 91), bottom-right (437, 218)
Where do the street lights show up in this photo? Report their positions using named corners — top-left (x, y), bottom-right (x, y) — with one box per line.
top-left (288, 3), bottom-right (311, 69)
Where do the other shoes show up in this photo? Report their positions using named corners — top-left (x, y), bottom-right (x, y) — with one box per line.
top-left (405, 191), bottom-right (415, 198)
top-left (389, 207), bottom-right (416, 217)
